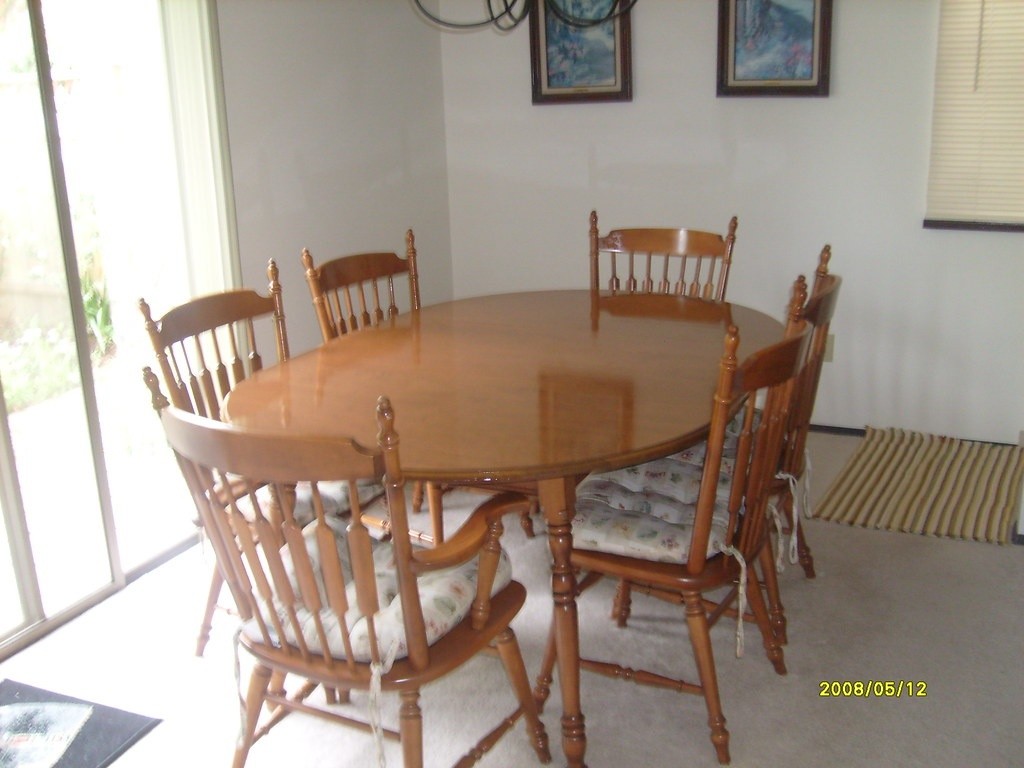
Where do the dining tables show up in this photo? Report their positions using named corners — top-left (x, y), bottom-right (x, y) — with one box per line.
top-left (222, 291), bottom-right (787, 768)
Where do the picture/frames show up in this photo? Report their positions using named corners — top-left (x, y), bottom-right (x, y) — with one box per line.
top-left (529, 0), bottom-right (633, 105)
top-left (716, 0), bottom-right (832, 98)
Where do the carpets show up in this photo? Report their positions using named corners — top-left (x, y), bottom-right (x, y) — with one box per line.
top-left (805, 425), bottom-right (1024, 545)
top-left (1, 678), bottom-right (163, 767)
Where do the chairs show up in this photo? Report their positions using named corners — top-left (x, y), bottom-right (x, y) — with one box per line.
top-left (300, 229), bottom-right (540, 545)
top-left (589, 210), bottom-right (739, 302)
top-left (528, 275), bottom-right (818, 768)
top-left (141, 366), bottom-right (555, 768)
top-left (609, 243), bottom-right (841, 645)
top-left (138, 257), bottom-right (392, 658)
